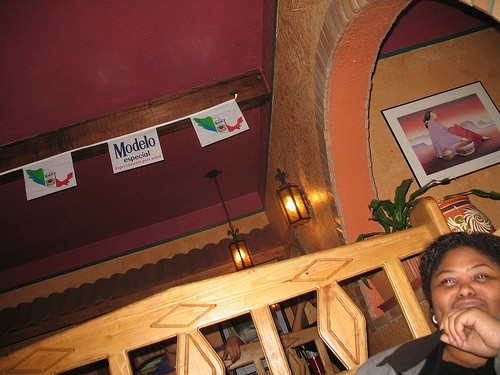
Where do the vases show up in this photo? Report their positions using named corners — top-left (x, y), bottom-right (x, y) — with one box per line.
top-left (436, 195), bottom-right (495, 234)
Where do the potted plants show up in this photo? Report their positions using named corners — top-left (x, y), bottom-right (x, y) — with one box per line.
top-left (357, 175), bottom-right (459, 313)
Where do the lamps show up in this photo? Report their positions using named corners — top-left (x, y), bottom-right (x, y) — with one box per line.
top-left (204, 170), bottom-right (254, 272)
top-left (274, 168), bottom-right (312, 228)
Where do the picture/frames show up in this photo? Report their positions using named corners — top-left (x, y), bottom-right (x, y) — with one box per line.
top-left (380, 81), bottom-right (500, 189)
